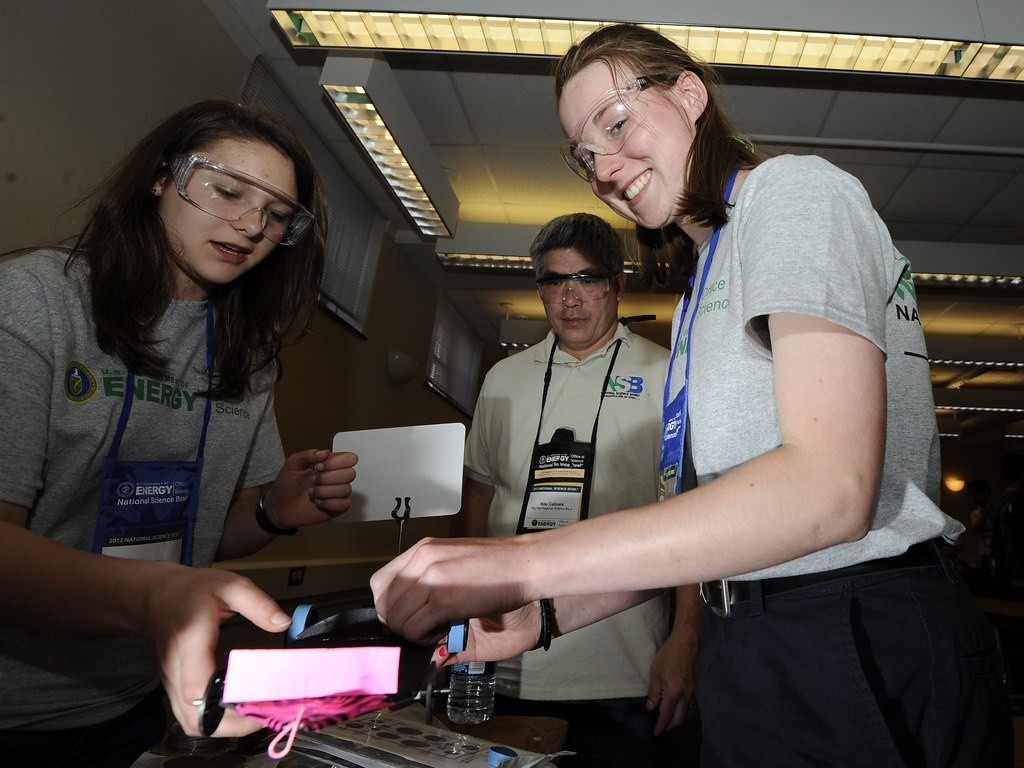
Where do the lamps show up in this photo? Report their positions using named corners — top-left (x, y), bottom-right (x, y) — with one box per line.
top-left (388, 349), bottom-right (420, 385)
top-left (268, 0), bottom-right (1024, 86)
top-left (319, 52), bottom-right (460, 240)
top-left (499, 301), bottom-right (1024, 371)
top-left (933, 386), bottom-right (1024, 412)
top-left (435, 220), bottom-right (1024, 296)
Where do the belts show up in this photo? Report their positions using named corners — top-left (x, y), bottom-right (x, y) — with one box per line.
top-left (700, 536), bottom-right (955, 617)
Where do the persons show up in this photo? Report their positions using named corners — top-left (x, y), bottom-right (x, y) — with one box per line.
top-left (369, 24), bottom-right (1018, 768)
top-left (463, 211), bottom-right (705, 768)
top-left (0, 98), bottom-right (358, 768)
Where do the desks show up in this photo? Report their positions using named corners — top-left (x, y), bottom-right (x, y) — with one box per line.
top-left (129, 714), bottom-right (570, 768)
top-left (209, 517), bottom-right (451, 572)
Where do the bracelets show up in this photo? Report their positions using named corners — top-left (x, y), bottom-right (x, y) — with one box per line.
top-left (255, 494), bottom-right (299, 537)
top-left (529, 598), bottom-right (552, 652)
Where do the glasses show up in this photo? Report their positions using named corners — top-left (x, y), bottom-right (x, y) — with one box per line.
top-left (535, 271), bottom-right (620, 303)
top-left (559, 71), bottom-right (680, 184)
top-left (153, 153), bottom-right (315, 246)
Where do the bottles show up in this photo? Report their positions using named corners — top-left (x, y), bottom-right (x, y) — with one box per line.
top-left (447, 661), bottom-right (497, 724)
